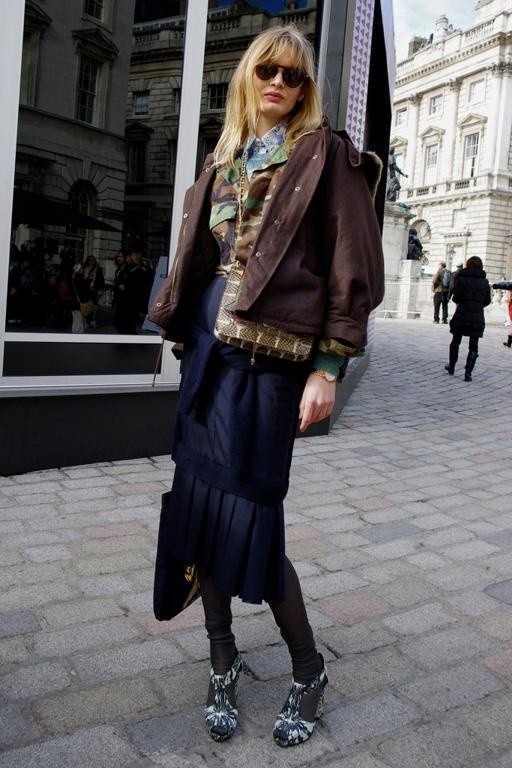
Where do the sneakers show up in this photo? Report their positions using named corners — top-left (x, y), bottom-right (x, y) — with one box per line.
top-left (433, 319), bottom-right (448, 324)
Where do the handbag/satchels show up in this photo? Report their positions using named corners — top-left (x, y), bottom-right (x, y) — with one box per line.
top-left (154, 490), bottom-right (202, 621)
top-left (214, 266), bottom-right (314, 361)
top-left (80, 301), bottom-right (95, 316)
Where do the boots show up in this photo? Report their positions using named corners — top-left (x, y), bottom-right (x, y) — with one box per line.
top-left (464, 351), bottom-right (478, 382)
top-left (445, 344), bottom-right (459, 376)
top-left (503, 335), bottom-right (512, 348)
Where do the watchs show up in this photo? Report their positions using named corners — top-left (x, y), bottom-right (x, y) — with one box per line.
top-left (313, 368), bottom-right (337, 384)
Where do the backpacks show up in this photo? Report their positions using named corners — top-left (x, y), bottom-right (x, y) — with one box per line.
top-left (438, 270), bottom-right (451, 288)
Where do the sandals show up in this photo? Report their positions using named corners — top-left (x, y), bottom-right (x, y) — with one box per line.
top-left (204, 652), bottom-right (243, 742)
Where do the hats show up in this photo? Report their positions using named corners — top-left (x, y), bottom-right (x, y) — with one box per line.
top-left (129, 251), bottom-right (143, 266)
top-left (74, 264), bottom-right (82, 273)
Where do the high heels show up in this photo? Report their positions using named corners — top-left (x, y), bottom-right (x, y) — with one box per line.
top-left (272, 652), bottom-right (328, 747)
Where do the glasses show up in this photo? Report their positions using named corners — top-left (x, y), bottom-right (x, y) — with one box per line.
top-left (255, 59), bottom-right (306, 88)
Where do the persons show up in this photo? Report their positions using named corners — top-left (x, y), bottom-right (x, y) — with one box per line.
top-left (432, 262), bottom-right (452, 324)
top-left (445, 256), bottom-right (491, 381)
top-left (450, 262), bottom-right (463, 298)
top-left (8, 245), bottom-right (156, 334)
top-left (488, 280), bottom-right (512, 348)
top-left (146, 23), bottom-right (386, 747)
top-left (408, 229), bottom-right (423, 259)
top-left (388, 147), bottom-right (409, 200)
